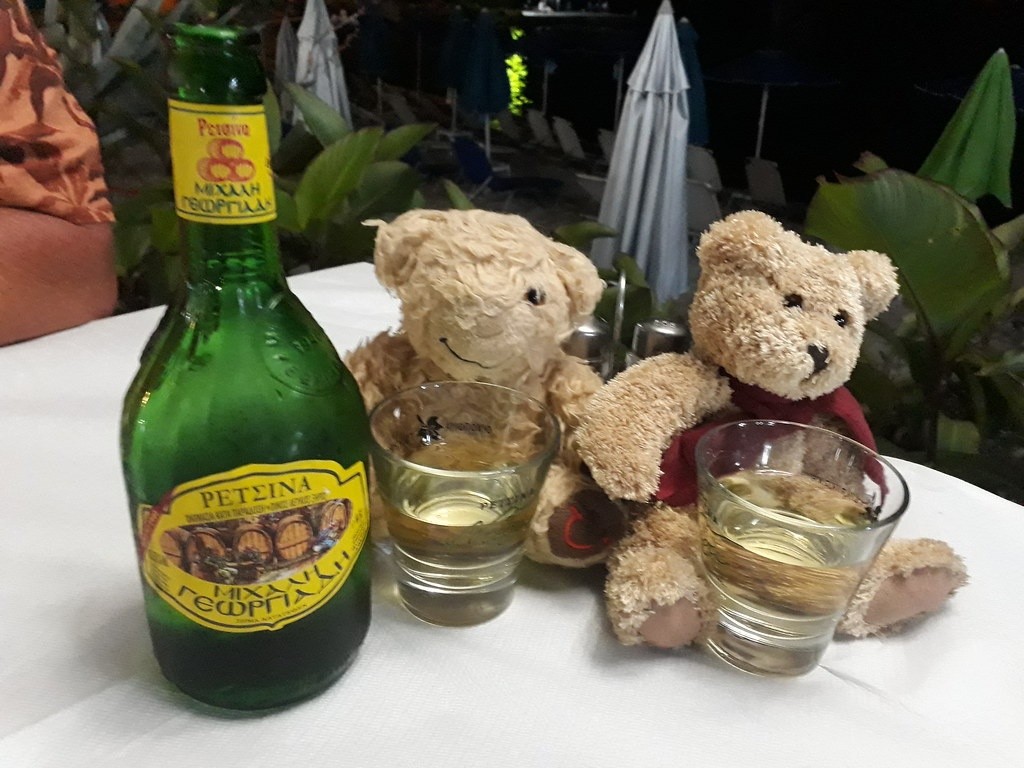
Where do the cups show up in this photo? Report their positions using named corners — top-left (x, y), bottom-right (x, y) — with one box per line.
top-left (366, 379), bottom-right (563, 630)
top-left (699, 416), bottom-right (908, 680)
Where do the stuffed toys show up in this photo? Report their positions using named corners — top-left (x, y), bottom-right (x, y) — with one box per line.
top-left (581, 209), bottom-right (968, 649)
top-left (344, 208), bottom-right (629, 566)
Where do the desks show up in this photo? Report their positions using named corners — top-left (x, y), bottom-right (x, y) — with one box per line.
top-left (1, 261), bottom-right (1024, 768)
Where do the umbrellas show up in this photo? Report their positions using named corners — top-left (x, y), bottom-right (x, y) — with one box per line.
top-left (291, 0), bottom-right (352, 133)
top-left (273, 18), bottom-right (297, 123)
top-left (592, 0), bottom-right (691, 341)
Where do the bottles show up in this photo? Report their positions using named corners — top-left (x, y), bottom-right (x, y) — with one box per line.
top-left (117, 20), bottom-right (373, 714)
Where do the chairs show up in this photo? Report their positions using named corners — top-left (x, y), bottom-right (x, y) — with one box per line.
top-left (376, 79), bottom-right (786, 249)
